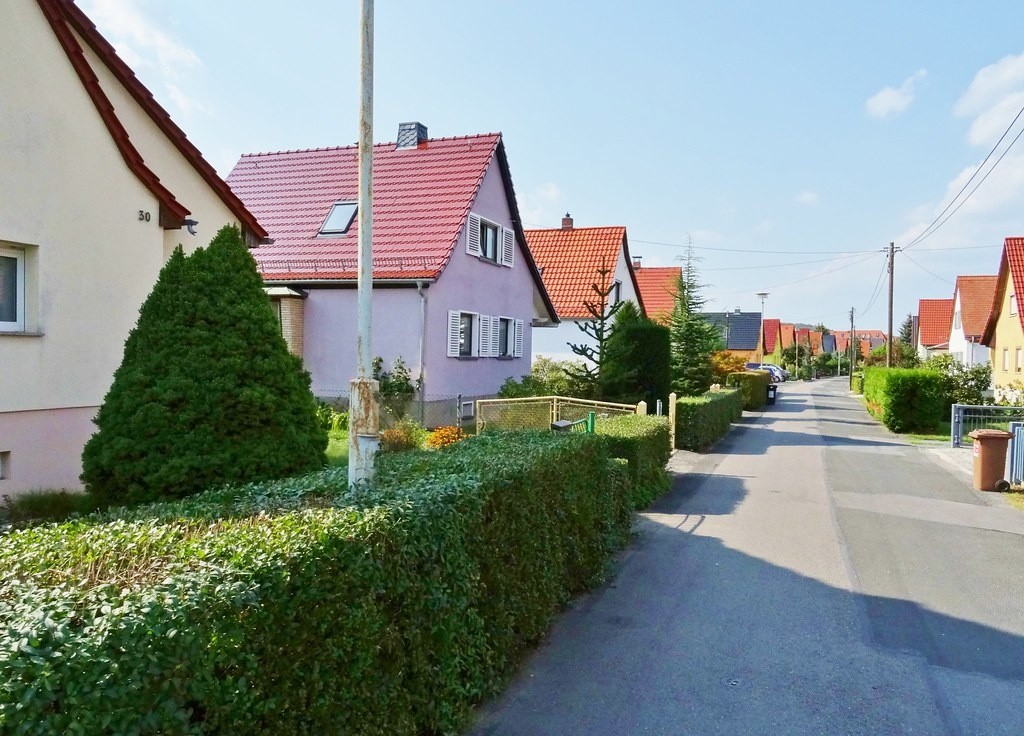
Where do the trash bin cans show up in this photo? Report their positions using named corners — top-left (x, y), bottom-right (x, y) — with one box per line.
top-left (816, 371), bottom-right (821, 379)
top-left (967, 429), bottom-right (1014, 492)
top-left (766, 384), bottom-right (778, 405)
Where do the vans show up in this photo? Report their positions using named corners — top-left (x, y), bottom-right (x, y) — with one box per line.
top-left (745, 362), bottom-right (790, 382)
